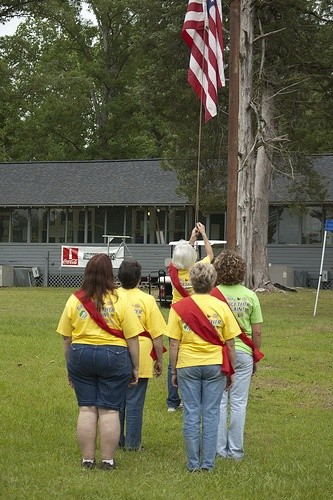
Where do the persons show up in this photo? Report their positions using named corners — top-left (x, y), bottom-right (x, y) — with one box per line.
top-left (209, 248), bottom-right (264, 464)
top-left (160, 260), bottom-right (243, 473)
top-left (113, 260), bottom-right (168, 454)
top-left (165, 222), bottom-right (215, 412)
top-left (56, 253), bottom-right (146, 471)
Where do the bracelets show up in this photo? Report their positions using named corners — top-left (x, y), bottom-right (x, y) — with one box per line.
top-left (170, 372), bottom-right (176, 375)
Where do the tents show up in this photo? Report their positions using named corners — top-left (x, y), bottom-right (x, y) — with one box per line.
top-left (312, 218), bottom-right (332, 316)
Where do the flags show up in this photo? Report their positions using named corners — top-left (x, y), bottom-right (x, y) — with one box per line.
top-left (180, 0), bottom-right (225, 123)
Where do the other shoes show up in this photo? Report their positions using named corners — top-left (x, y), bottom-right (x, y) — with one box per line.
top-left (82, 461), bottom-right (96, 469)
top-left (168, 407), bottom-right (176, 411)
top-left (99, 463), bottom-right (116, 470)
